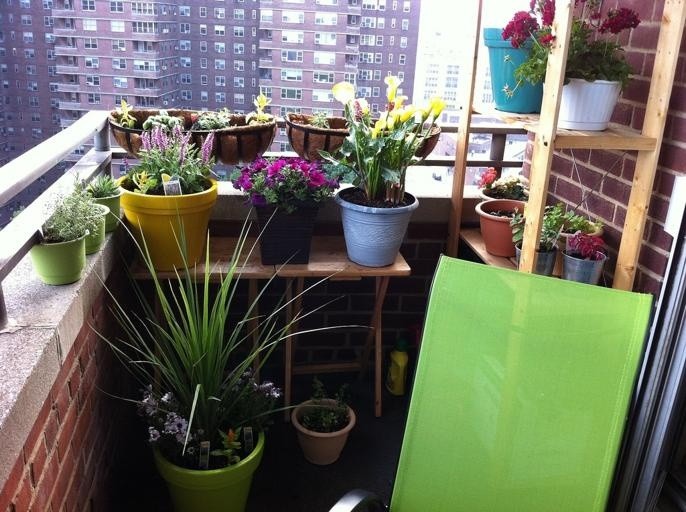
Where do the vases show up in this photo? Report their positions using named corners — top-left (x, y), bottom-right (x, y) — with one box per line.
top-left (253, 196), bottom-right (320, 266)
top-left (334, 187), bottom-right (420, 266)
top-left (484, 26), bottom-right (554, 114)
top-left (149, 432), bottom-right (266, 511)
top-left (117, 171), bottom-right (217, 272)
top-left (543, 75), bottom-right (625, 131)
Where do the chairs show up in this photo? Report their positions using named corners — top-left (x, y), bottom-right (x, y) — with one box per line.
top-left (327, 250), bottom-right (654, 511)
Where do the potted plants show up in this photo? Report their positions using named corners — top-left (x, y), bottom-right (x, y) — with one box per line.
top-left (368, 121), bottom-right (444, 160)
top-left (291, 375), bottom-right (358, 465)
top-left (26, 174), bottom-right (123, 283)
top-left (283, 110), bottom-right (352, 160)
top-left (474, 163), bottom-right (609, 285)
top-left (107, 105), bottom-right (279, 162)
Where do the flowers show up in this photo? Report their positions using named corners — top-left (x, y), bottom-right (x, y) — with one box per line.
top-left (500, 0), bottom-right (642, 99)
top-left (228, 155), bottom-right (345, 210)
top-left (310, 75), bottom-right (446, 206)
top-left (75, 195), bottom-right (377, 468)
top-left (120, 123), bottom-right (217, 193)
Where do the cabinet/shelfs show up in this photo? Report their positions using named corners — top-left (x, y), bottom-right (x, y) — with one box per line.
top-left (444, 2), bottom-right (686, 293)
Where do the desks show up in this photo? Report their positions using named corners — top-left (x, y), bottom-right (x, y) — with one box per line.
top-left (277, 231), bottom-right (414, 420)
top-left (127, 233), bottom-right (276, 395)
